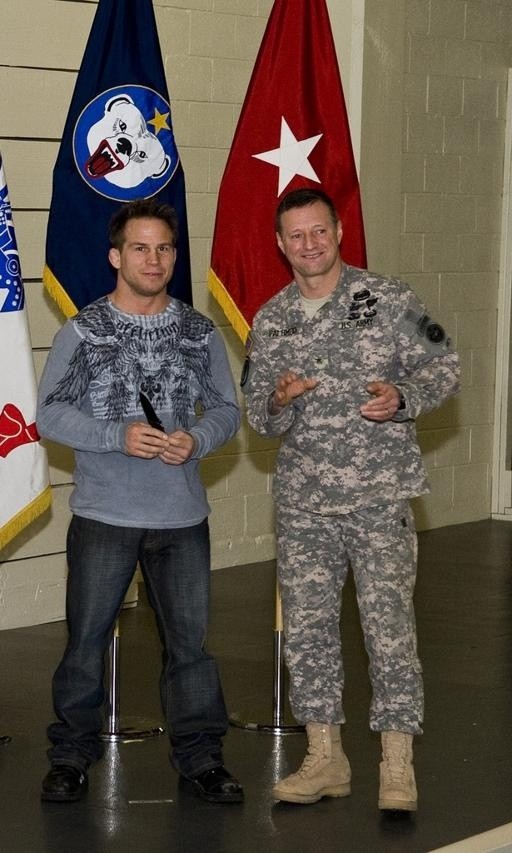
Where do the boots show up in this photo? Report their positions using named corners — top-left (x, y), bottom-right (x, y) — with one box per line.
top-left (378, 731), bottom-right (418, 811)
top-left (272, 721), bottom-right (351, 803)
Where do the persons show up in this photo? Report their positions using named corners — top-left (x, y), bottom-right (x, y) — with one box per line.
top-left (243, 186), bottom-right (463, 814)
top-left (34, 197), bottom-right (245, 806)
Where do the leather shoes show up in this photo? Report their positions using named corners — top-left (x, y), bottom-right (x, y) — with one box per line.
top-left (178, 766), bottom-right (243, 801)
top-left (40, 758), bottom-right (88, 801)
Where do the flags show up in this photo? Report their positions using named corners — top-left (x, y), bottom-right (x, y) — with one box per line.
top-left (203, 1), bottom-right (369, 354)
top-left (2, 160), bottom-right (57, 564)
top-left (40, 1), bottom-right (193, 330)
